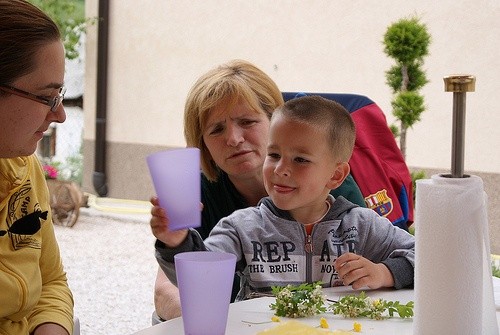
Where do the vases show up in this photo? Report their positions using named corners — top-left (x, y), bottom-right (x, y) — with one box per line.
top-left (47, 178), bottom-right (71, 201)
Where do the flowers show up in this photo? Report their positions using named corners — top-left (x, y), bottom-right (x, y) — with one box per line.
top-left (348, 322), bottom-right (362, 332)
top-left (242, 315), bottom-right (280, 324)
top-left (269, 281), bottom-right (414, 321)
top-left (39, 153), bottom-right (81, 182)
top-left (316, 318), bottom-right (329, 329)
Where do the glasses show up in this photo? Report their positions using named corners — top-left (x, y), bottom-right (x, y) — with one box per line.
top-left (0, 83), bottom-right (67, 112)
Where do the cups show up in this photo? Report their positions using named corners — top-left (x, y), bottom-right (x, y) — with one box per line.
top-left (174, 251), bottom-right (237, 334)
top-left (146, 147), bottom-right (201, 230)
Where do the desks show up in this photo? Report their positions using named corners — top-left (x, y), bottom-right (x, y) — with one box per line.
top-left (134, 275), bottom-right (500, 335)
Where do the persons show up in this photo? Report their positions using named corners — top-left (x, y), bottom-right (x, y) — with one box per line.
top-left (0, 0), bottom-right (75, 335)
top-left (155, 58), bottom-right (367, 320)
top-left (149, 94), bottom-right (415, 302)
